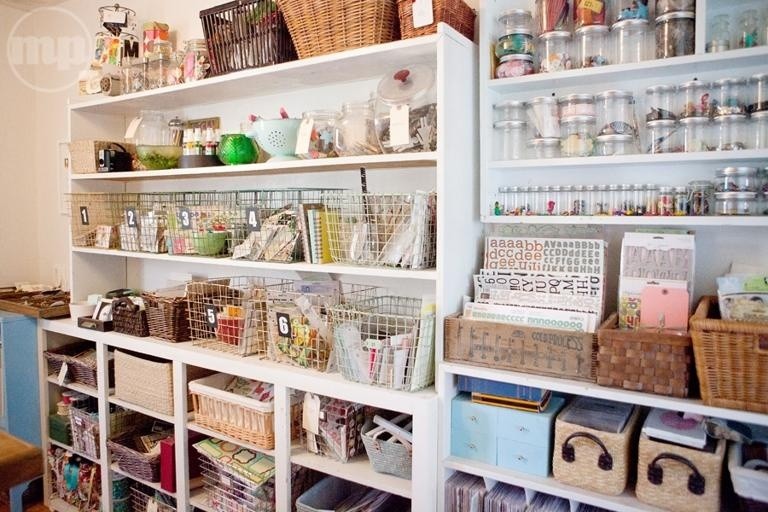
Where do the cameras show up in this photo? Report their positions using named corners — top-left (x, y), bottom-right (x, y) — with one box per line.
top-left (98, 147), bottom-right (133, 172)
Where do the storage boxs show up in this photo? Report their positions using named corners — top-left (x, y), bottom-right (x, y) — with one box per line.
top-left (451, 398), bottom-right (564, 478)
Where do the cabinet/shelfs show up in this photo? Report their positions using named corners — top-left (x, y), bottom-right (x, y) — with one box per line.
top-left (438, 361), bottom-right (767, 511)
top-left (479, 0), bottom-right (768, 227)
top-left (0, 308), bottom-right (36, 512)
top-left (36, 318), bottom-right (438, 512)
top-left (67, 22), bottom-right (481, 395)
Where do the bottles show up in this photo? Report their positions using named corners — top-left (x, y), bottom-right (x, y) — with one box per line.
top-left (594, 134), bottom-right (636, 157)
top-left (657, 187), bottom-right (675, 216)
top-left (761, 167), bottom-right (768, 192)
top-left (609, 184), bottom-right (622, 216)
top-left (493, 120), bottom-right (527, 163)
top-left (558, 115), bottom-right (599, 158)
top-left (645, 183), bottom-right (658, 215)
top-left (714, 166), bottom-right (759, 192)
top-left (491, 99), bottom-right (525, 120)
top-left (573, 184), bottom-right (586, 216)
top-left (373, 64), bottom-right (435, 155)
top-left (678, 80), bottom-right (710, 120)
top-left (539, 185), bottom-right (550, 216)
top-left (297, 110), bottom-right (336, 159)
top-left (571, 0), bottom-right (609, 30)
top-left (653, 0), bottom-right (696, 16)
top-left (496, 8), bottom-right (533, 35)
top-left (738, 9), bottom-right (759, 49)
top-left (644, 119), bottom-right (678, 154)
top-left (525, 138), bottom-right (561, 159)
top-left (654, 11), bottom-right (695, 60)
top-left (634, 183), bottom-right (646, 216)
top-left (598, 183), bottom-right (609, 216)
top-left (533, 0), bottom-right (571, 35)
top-left (592, 90), bottom-right (638, 135)
top-left (330, 103), bottom-right (381, 158)
top-left (678, 118), bottom-right (709, 153)
top-left (573, 25), bottom-right (611, 69)
top-left (611, 18), bottom-right (652, 66)
top-left (495, 30), bottom-right (536, 59)
top-left (750, 111), bottom-right (768, 151)
top-left (130, 56), bottom-right (147, 94)
top-left (528, 185), bottom-right (539, 216)
top-left (586, 184), bottom-right (598, 216)
top-left (508, 185), bottom-right (518, 216)
top-left (687, 179), bottom-right (713, 217)
top-left (609, 0), bottom-right (653, 24)
top-left (152, 40), bottom-right (172, 53)
top-left (150, 55), bottom-right (169, 91)
top-left (712, 192), bottom-right (760, 214)
top-left (713, 115), bottom-right (748, 152)
top-left (560, 185), bottom-right (574, 216)
top-left (761, 192), bottom-right (768, 215)
top-left (674, 184), bottom-right (688, 216)
top-left (523, 96), bottom-right (560, 139)
top-left (535, 30), bottom-right (573, 74)
top-left (620, 184), bottom-right (633, 216)
top-left (706, 13), bottom-right (731, 53)
top-left (549, 185), bottom-right (560, 216)
top-left (557, 93), bottom-right (598, 117)
top-left (498, 185), bottom-right (508, 216)
top-left (518, 185), bottom-right (528, 216)
top-left (748, 72), bottom-right (767, 114)
top-left (712, 75), bottom-right (747, 115)
top-left (645, 85), bottom-right (676, 121)
top-left (494, 54), bottom-right (535, 78)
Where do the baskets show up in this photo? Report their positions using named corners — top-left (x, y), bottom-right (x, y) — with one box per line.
top-left (276, 0), bottom-right (396, 60)
top-left (396, 0), bottom-right (476, 43)
top-left (143, 299), bottom-right (189, 343)
top-left (106, 422), bottom-right (159, 482)
top-left (596, 312), bottom-right (689, 397)
top-left (111, 297), bottom-right (149, 337)
top-left (188, 372), bottom-right (304, 450)
top-left (688, 293), bottom-right (768, 415)
top-left (42, 341), bottom-right (97, 387)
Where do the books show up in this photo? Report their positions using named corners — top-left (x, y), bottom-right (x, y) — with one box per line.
top-left (645, 409), bottom-right (709, 448)
top-left (298, 202), bottom-right (342, 264)
top-left (563, 396), bottom-right (634, 433)
